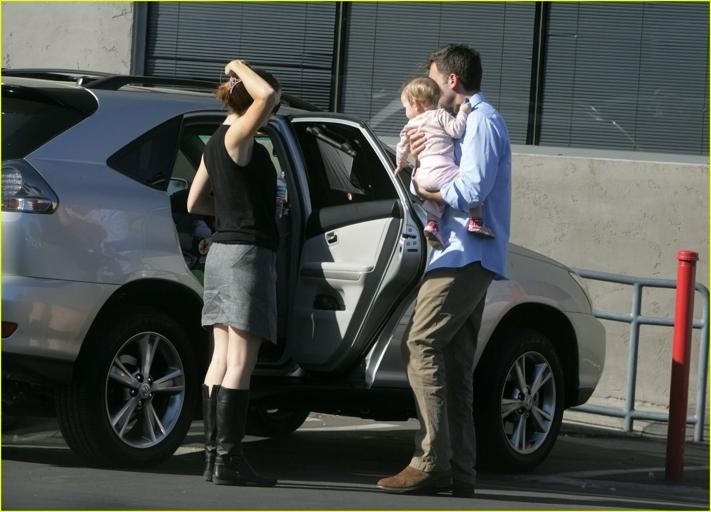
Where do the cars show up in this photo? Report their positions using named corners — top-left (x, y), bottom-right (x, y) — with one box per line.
top-left (1, 68), bottom-right (605, 470)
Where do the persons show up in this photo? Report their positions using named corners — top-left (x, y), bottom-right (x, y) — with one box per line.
top-left (377, 43), bottom-right (511, 496)
top-left (186, 59), bottom-right (282, 487)
top-left (393, 77), bottom-right (496, 247)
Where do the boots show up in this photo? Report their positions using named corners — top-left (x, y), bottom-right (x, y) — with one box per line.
top-left (201, 383), bottom-right (279, 487)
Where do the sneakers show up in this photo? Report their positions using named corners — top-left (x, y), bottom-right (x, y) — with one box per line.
top-left (468, 219), bottom-right (495, 240)
top-left (423, 225), bottom-right (445, 251)
top-left (376, 464), bottom-right (477, 498)
